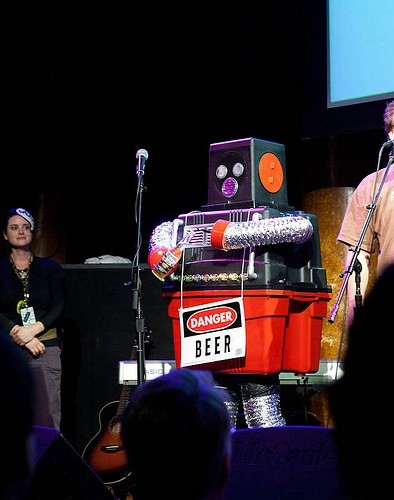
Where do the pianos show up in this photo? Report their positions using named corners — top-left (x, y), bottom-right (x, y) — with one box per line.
top-left (115, 359), bottom-right (344, 388)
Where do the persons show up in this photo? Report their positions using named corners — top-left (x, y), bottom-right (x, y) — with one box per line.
top-left (121, 369), bottom-right (232, 500)
top-left (0, 208), bottom-right (66, 434)
top-left (337, 100), bottom-right (394, 311)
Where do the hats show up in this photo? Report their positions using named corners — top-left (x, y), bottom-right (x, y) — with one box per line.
top-left (5, 208), bottom-right (36, 231)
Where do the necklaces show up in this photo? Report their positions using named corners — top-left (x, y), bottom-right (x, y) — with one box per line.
top-left (10, 253), bottom-right (31, 314)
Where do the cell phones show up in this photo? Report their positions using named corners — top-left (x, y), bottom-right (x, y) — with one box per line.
top-left (20, 306), bottom-right (36, 326)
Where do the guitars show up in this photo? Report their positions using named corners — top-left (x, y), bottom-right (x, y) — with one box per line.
top-left (82, 337), bottom-right (138, 485)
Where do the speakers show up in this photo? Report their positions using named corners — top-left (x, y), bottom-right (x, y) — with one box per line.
top-left (207, 138), bottom-right (289, 208)
top-left (29, 425), bottom-right (117, 500)
top-left (223, 424), bottom-right (341, 500)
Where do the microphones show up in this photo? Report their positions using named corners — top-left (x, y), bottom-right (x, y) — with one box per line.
top-left (135, 149), bottom-right (149, 180)
top-left (382, 139), bottom-right (394, 147)
